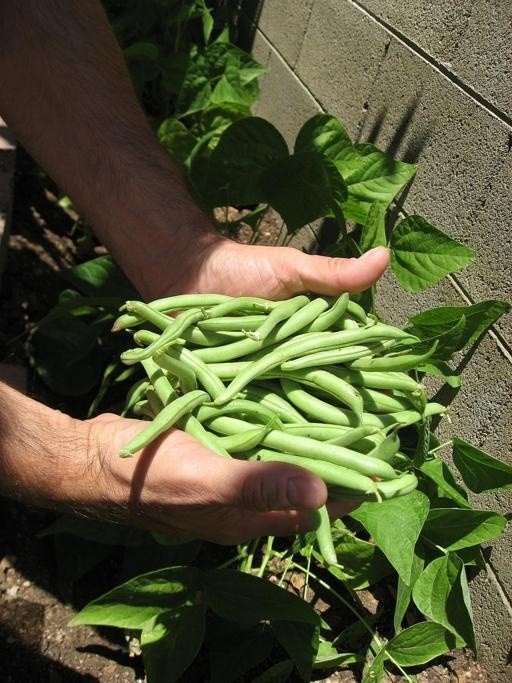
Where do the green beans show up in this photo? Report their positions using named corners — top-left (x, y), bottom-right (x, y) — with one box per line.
top-left (109, 288), bottom-right (446, 570)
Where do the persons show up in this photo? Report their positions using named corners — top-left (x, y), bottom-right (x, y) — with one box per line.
top-left (0, 0), bottom-right (391, 550)
